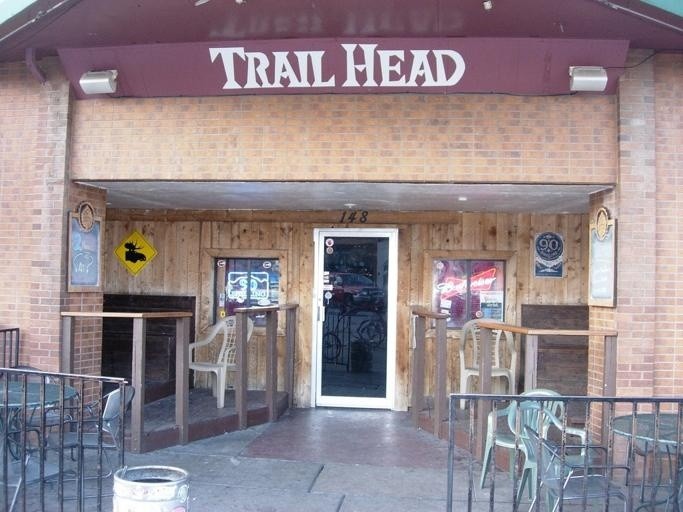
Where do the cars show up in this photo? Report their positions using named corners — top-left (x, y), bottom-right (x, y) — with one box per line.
top-left (329, 272), bottom-right (385, 315)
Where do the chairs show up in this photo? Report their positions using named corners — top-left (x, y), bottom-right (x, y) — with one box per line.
top-left (1, 364), bottom-right (136, 502)
top-left (479, 387), bottom-right (635, 511)
top-left (188, 313), bottom-right (255, 409)
top-left (457, 318), bottom-right (518, 410)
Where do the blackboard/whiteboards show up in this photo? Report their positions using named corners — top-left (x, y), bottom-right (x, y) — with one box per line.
top-left (67, 211), bottom-right (105, 292)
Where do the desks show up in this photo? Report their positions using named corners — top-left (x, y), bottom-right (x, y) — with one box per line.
top-left (607, 412), bottom-right (683, 512)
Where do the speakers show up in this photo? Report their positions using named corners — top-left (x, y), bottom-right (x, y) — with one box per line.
top-left (79, 72), bottom-right (117, 95)
top-left (570, 67), bottom-right (609, 93)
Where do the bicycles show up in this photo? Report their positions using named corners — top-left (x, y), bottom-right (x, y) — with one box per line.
top-left (323, 304), bottom-right (341, 360)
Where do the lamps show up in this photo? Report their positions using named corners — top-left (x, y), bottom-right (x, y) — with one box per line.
top-left (567, 64), bottom-right (609, 93)
top-left (78, 67), bottom-right (118, 97)
